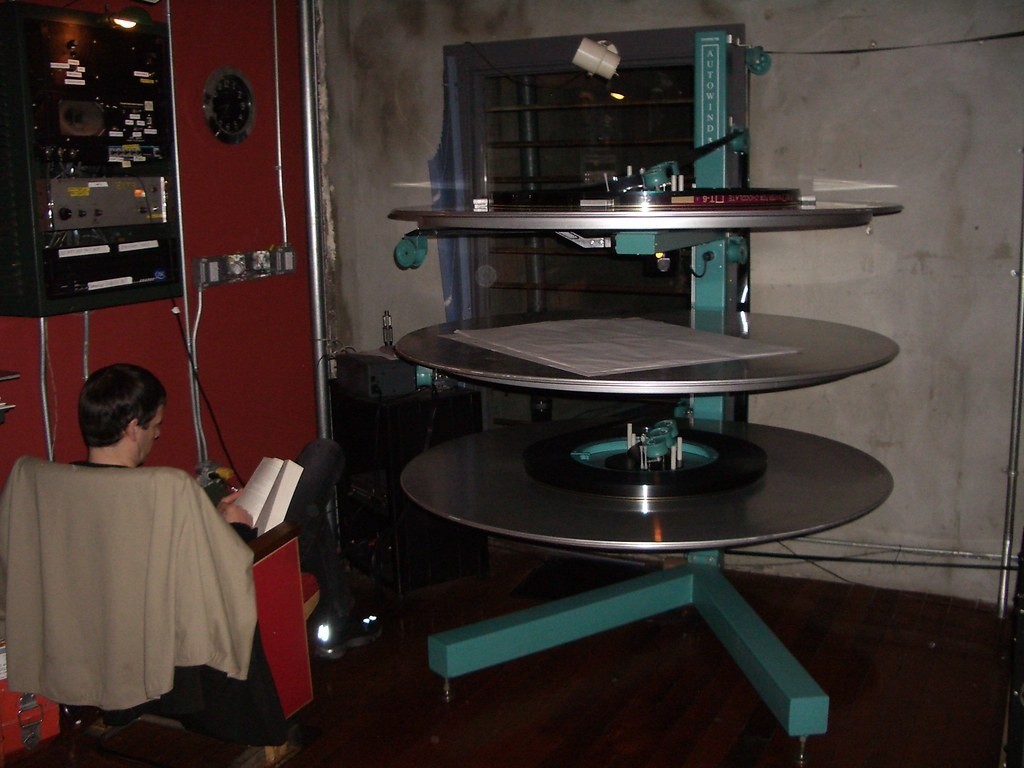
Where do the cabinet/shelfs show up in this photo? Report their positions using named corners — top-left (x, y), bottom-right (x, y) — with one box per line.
top-left (328, 379), bottom-right (489, 592)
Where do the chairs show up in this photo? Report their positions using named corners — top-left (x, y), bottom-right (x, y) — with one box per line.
top-left (0, 454), bottom-right (332, 768)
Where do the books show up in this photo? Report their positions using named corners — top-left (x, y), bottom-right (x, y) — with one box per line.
top-left (234, 456), bottom-right (304, 537)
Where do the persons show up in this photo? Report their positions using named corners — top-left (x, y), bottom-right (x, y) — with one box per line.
top-left (68, 363), bottom-right (383, 660)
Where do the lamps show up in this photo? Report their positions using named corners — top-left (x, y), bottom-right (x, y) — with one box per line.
top-left (573, 37), bottom-right (621, 80)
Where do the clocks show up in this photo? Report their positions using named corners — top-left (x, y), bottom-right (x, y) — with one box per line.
top-left (201, 65), bottom-right (258, 143)
top-left (112, 6), bottom-right (154, 29)
